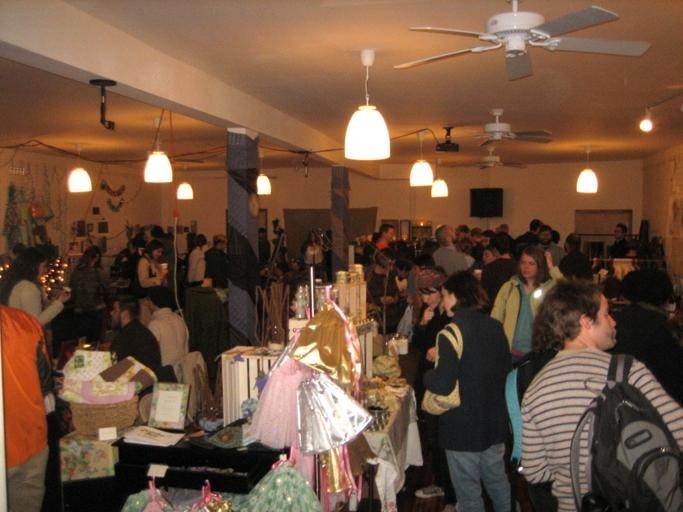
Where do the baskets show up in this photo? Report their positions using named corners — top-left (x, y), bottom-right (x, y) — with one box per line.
top-left (70, 395), bottom-right (138, 435)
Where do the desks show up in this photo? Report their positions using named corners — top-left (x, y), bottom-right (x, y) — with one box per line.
top-left (223, 345), bottom-right (292, 424)
top-left (59, 415), bottom-right (289, 508)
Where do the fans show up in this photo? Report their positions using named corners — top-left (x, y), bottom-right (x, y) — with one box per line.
top-left (439, 108), bottom-right (552, 148)
top-left (392, 1), bottom-right (655, 78)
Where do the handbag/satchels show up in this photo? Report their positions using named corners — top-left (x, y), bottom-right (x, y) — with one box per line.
top-left (421, 323), bottom-right (464, 416)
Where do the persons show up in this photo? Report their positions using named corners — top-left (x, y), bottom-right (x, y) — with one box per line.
top-left (520, 276), bottom-right (683, 511)
top-left (1, 303), bottom-right (53, 511)
top-left (422, 271), bottom-right (515, 511)
top-left (1, 219), bottom-right (683, 511)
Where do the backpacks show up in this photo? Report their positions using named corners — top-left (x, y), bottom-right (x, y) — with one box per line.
top-left (570, 352), bottom-right (682, 511)
